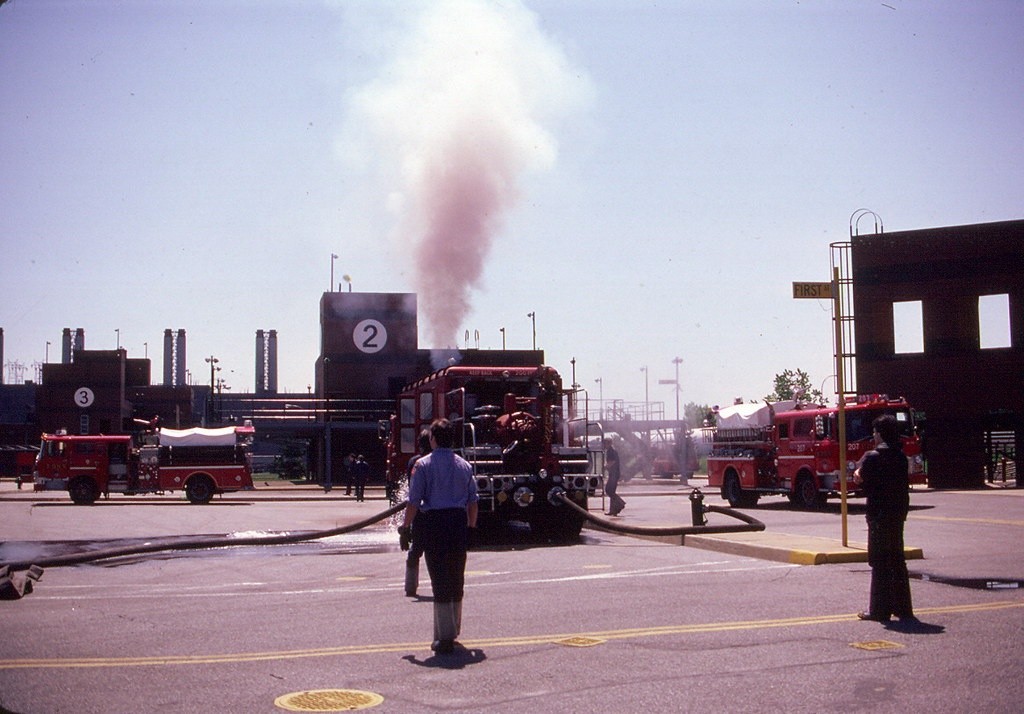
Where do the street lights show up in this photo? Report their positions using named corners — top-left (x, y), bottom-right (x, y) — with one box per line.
top-left (331, 252), bottom-right (340, 291)
top-left (186, 367), bottom-right (194, 387)
top-left (671, 357), bottom-right (683, 422)
top-left (206, 354), bottom-right (232, 426)
top-left (570, 356), bottom-right (577, 388)
top-left (143, 341), bottom-right (149, 361)
top-left (526, 311), bottom-right (537, 349)
top-left (114, 327), bottom-right (120, 349)
top-left (594, 377), bottom-right (603, 423)
top-left (639, 364), bottom-right (650, 423)
top-left (45, 342), bottom-right (52, 365)
top-left (498, 327), bottom-right (507, 349)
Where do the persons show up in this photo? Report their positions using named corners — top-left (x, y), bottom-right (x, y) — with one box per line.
top-left (853, 414), bottom-right (913, 619)
top-left (604, 438), bottom-right (626, 517)
top-left (343, 429), bottom-right (432, 596)
top-left (400, 419), bottom-right (480, 652)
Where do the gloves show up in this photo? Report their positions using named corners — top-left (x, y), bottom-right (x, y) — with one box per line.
top-left (398, 526), bottom-right (412, 551)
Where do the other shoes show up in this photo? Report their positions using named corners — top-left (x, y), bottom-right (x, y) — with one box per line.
top-left (894, 613), bottom-right (911, 620)
top-left (618, 505), bottom-right (625, 513)
top-left (857, 611), bottom-right (889, 623)
top-left (343, 493), bottom-right (350, 496)
top-left (604, 511), bottom-right (613, 515)
top-left (431, 638), bottom-right (454, 653)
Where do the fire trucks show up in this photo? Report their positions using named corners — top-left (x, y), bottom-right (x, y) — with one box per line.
top-left (644, 440), bottom-right (701, 480)
top-left (707, 393), bottom-right (928, 512)
top-left (29, 413), bottom-right (257, 503)
top-left (377, 358), bottom-right (608, 543)
